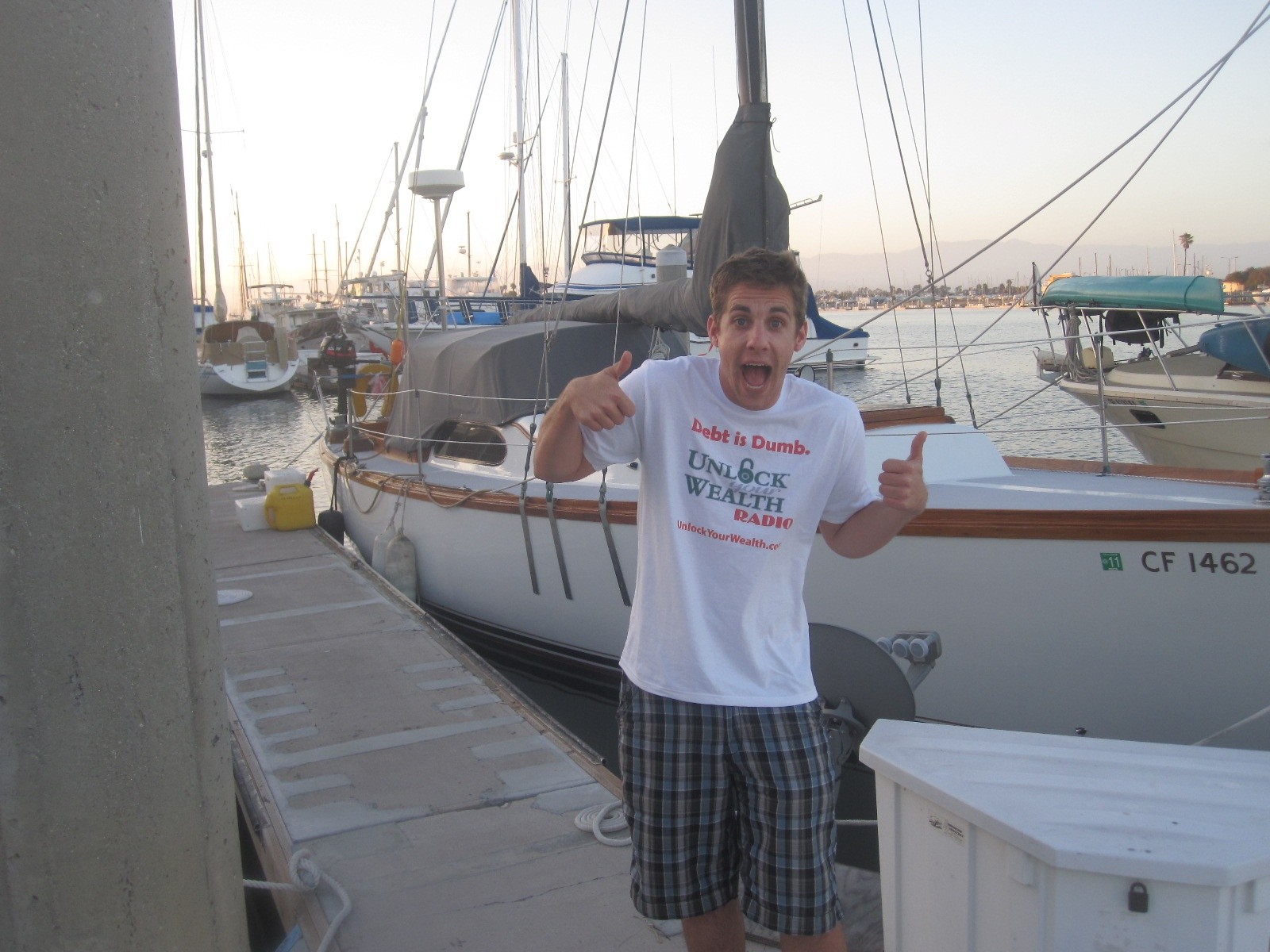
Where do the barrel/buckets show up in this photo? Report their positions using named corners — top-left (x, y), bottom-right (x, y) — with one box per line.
top-left (265, 468), bottom-right (317, 531)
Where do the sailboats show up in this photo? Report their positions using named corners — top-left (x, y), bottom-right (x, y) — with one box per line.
top-left (190, 0), bottom-right (871, 399)
top-left (314, 0), bottom-right (1269, 878)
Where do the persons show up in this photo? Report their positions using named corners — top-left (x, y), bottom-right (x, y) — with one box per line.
top-left (533, 247), bottom-right (929, 951)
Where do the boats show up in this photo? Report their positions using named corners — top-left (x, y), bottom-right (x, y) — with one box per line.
top-left (1198, 318), bottom-right (1270, 377)
top-left (1039, 276), bottom-right (1224, 313)
top-left (1031, 313), bottom-right (1269, 469)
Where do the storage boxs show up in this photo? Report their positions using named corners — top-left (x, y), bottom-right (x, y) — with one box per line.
top-left (235, 496), bottom-right (269, 531)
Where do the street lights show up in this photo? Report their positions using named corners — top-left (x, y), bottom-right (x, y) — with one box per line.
top-left (1221, 256), bottom-right (1237, 272)
